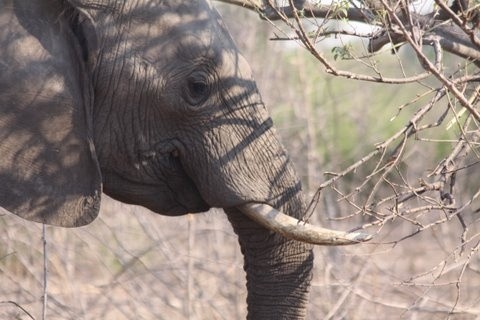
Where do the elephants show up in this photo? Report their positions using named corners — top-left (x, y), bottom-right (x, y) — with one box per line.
top-left (1, 0), bottom-right (374, 315)
